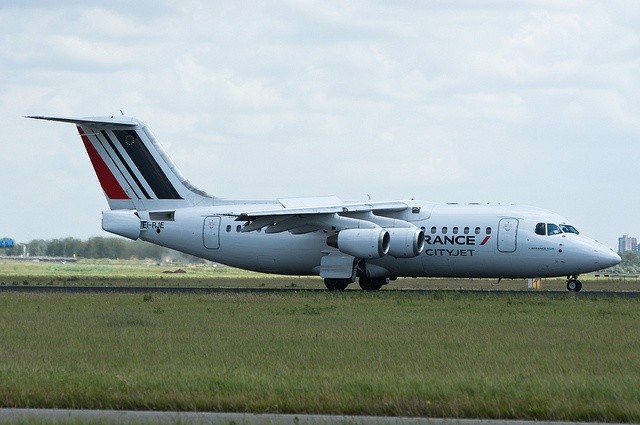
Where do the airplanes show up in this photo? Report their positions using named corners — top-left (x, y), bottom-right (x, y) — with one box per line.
top-left (23, 109), bottom-right (621, 291)
